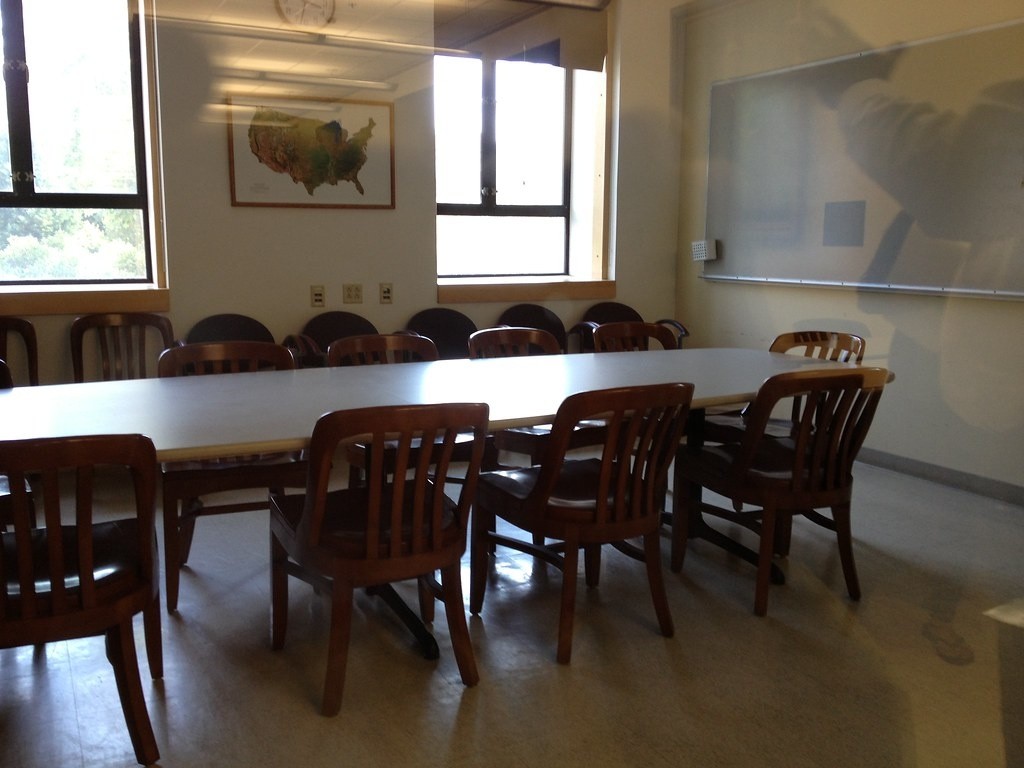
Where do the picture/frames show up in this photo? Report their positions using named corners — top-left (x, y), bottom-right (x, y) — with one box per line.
top-left (227, 91), bottom-right (397, 208)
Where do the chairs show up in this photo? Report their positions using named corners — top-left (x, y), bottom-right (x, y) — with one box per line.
top-left (0, 304), bottom-right (886, 765)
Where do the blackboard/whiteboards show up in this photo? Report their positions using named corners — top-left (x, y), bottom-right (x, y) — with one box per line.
top-left (697, 17), bottom-right (1024, 302)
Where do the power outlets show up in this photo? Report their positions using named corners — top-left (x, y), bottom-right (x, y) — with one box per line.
top-left (343, 284), bottom-right (364, 304)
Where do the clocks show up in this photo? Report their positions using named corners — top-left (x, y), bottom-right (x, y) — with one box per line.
top-left (275, 0), bottom-right (337, 30)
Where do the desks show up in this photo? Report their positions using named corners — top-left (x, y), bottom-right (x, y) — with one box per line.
top-left (0, 346), bottom-right (897, 588)
